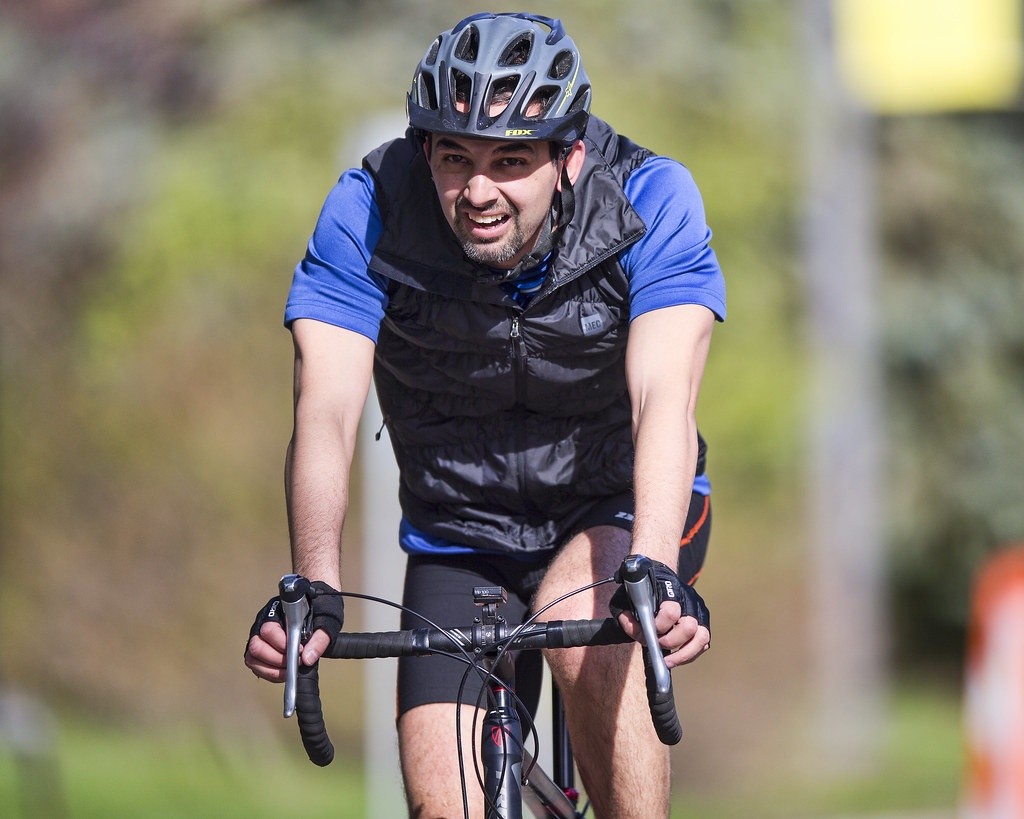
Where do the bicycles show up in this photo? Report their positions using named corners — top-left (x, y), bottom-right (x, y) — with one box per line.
top-left (282, 557), bottom-right (682, 819)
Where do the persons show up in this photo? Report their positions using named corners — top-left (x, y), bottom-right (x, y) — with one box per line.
top-left (244, 12), bottom-right (727, 819)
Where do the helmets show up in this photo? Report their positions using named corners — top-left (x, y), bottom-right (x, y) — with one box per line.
top-left (407, 11), bottom-right (595, 146)
